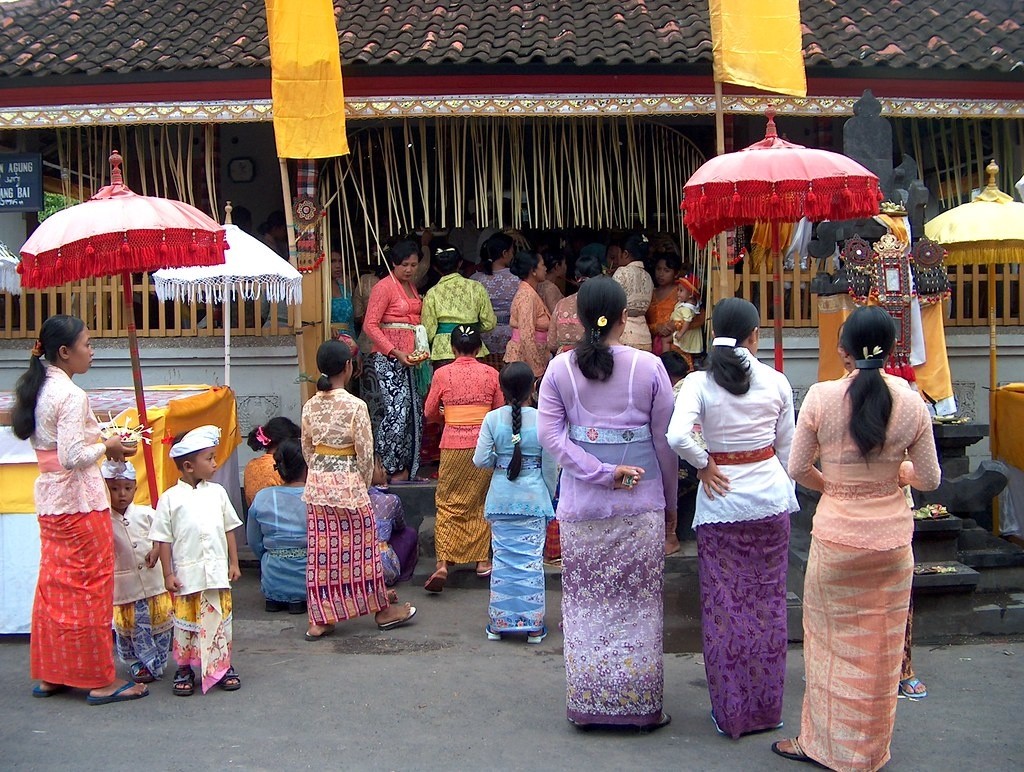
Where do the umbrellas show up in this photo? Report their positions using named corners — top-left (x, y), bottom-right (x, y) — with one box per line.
top-left (0, 240), bottom-right (23, 299)
top-left (920, 161), bottom-right (1024, 552)
top-left (150, 199), bottom-right (302, 387)
top-left (680, 103), bottom-right (877, 380)
top-left (17, 148), bottom-right (230, 510)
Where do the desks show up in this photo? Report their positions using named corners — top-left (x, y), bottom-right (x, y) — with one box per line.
top-left (0, 381), bottom-right (236, 642)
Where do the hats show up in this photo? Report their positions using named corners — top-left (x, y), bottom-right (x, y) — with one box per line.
top-left (675, 272), bottom-right (701, 299)
top-left (100, 459), bottom-right (136, 481)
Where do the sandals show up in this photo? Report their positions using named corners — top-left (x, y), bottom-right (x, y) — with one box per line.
top-left (130, 660), bottom-right (154, 683)
top-left (171, 667), bottom-right (196, 695)
top-left (217, 665), bottom-right (241, 690)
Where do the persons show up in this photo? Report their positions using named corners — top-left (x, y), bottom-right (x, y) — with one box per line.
top-left (99, 458), bottom-right (176, 685)
top-left (300, 339), bottom-right (418, 640)
top-left (243, 233), bottom-right (926, 704)
top-left (537, 274), bottom-right (678, 729)
top-left (473, 360), bottom-right (555, 644)
top-left (11, 316), bottom-right (149, 705)
top-left (769, 306), bottom-right (942, 772)
top-left (666, 296), bottom-right (799, 736)
top-left (150, 424), bottom-right (241, 697)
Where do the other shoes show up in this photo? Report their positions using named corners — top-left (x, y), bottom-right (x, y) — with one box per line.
top-left (265, 601), bottom-right (288, 611)
top-left (286, 601), bottom-right (308, 614)
top-left (769, 721), bottom-right (784, 729)
top-left (710, 710), bottom-right (727, 734)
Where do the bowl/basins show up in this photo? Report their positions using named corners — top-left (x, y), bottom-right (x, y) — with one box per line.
top-left (101, 427), bottom-right (142, 447)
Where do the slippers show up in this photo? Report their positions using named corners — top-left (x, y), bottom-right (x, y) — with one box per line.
top-left (31, 684), bottom-right (73, 697)
top-left (771, 736), bottom-right (827, 769)
top-left (424, 574), bottom-right (447, 593)
top-left (304, 624), bottom-right (337, 640)
top-left (566, 716), bottom-right (590, 730)
top-left (477, 569), bottom-right (492, 578)
top-left (390, 474), bottom-right (430, 484)
top-left (485, 625), bottom-right (501, 640)
top-left (900, 676), bottom-right (927, 698)
top-left (637, 711), bottom-right (672, 734)
top-left (542, 559), bottom-right (562, 567)
top-left (87, 679), bottom-right (150, 706)
top-left (379, 603), bottom-right (417, 630)
top-left (527, 625), bottom-right (548, 644)
